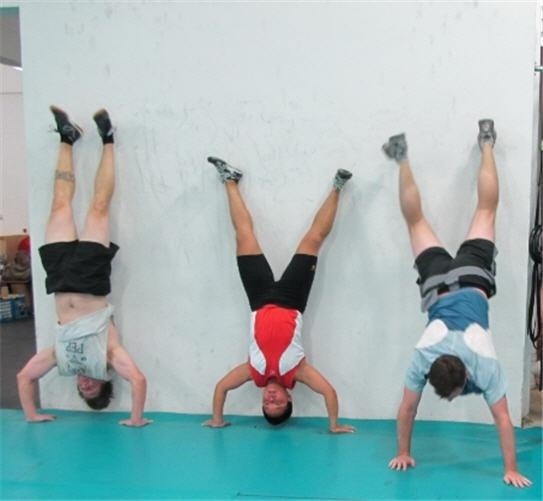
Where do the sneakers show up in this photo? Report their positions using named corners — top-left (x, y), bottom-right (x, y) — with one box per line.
top-left (333, 167), bottom-right (351, 191)
top-left (208, 157), bottom-right (243, 183)
top-left (476, 119), bottom-right (496, 147)
top-left (382, 133), bottom-right (409, 161)
top-left (95, 109), bottom-right (116, 136)
top-left (50, 104), bottom-right (83, 141)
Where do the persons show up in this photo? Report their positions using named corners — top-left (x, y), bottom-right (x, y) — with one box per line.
top-left (14, 102), bottom-right (151, 429)
top-left (198, 154), bottom-right (359, 436)
top-left (374, 116), bottom-right (534, 490)
